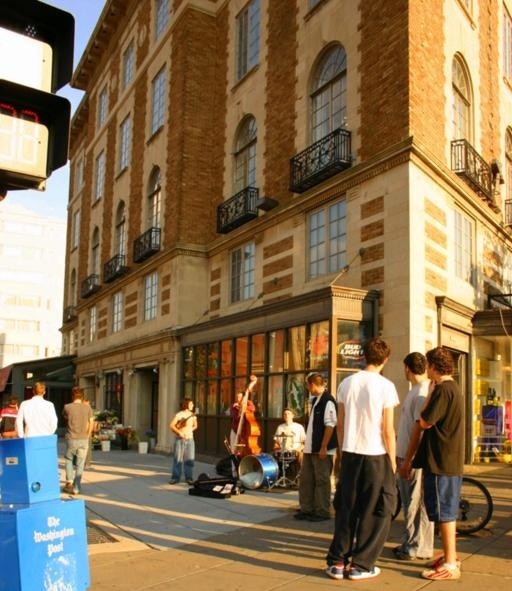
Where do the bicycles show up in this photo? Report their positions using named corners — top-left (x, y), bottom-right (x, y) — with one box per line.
top-left (390, 477), bottom-right (494, 535)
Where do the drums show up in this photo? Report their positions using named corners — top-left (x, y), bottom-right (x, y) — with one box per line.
top-left (238, 454), bottom-right (279, 489)
top-left (275, 450), bottom-right (297, 461)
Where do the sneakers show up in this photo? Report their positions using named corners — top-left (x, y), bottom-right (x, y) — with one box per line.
top-left (294, 512), bottom-right (327, 521)
top-left (326, 545), bottom-right (460, 579)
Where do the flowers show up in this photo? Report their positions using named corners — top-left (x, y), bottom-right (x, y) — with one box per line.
top-left (99, 435), bottom-right (110, 441)
top-left (117, 426), bottom-right (133, 435)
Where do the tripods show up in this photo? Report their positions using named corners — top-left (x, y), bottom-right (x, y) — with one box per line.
top-left (267, 459), bottom-right (300, 493)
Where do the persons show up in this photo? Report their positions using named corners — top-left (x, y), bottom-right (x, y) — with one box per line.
top-left (273, 408), bottom-right (306, 467)
top-left (0, 381), bottom-right (93, 494)
top-left (392, 353), bottom-right (434, 561)
top-left (230, 390), bottom-right (256, 478)
top-left (411, 347), bottom-right (465, 580)
top-left (294, 371), bottom-right (338, 522)
top-left (169, 399), bottom-right (197, 484)
top-left (326, 338), bottom-right (401, 580)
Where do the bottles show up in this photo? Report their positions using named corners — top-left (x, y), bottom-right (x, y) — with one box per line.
top-left (486, 388), bottom-right (501, 406)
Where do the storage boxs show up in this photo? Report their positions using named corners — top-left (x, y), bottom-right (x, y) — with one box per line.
top-left (0, 498), bottom-right (90, 591)
top-left (0, 434), bottom-right (60, 503)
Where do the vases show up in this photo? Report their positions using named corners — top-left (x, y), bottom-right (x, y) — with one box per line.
top-left (121, 435), bottom-right (130, 449)
top-left (101, 441), bottom-right (110, 452)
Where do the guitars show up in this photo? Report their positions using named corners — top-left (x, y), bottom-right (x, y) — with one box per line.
top-left (175, 412), bottom-right (196, 429)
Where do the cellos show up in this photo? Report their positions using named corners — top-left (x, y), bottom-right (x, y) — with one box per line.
top-left (233, 375), bottom-right (263, 461)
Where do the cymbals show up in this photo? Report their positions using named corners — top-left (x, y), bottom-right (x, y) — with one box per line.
top-left (275, 433), bottom-right (294, 437)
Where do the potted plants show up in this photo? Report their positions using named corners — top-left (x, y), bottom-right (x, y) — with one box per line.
top-left (131, 431), bottom-right (152, 454)
